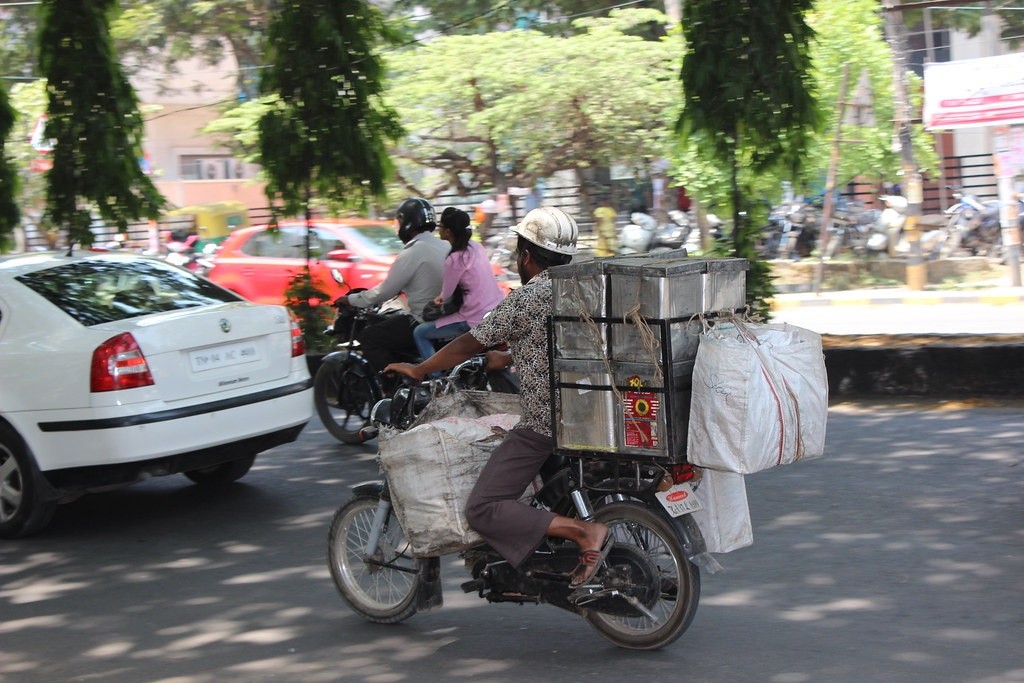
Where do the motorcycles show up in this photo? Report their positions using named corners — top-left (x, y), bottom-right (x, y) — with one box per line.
top-left (862, 189), bottom-right (950, 262)
top-left (314, 268), bottom-right (523, 444)
top-left (327, 353), bottom-right (707, 652)
top-left (622, 178), bottom-right (863, 265)
top-left (938, 183), bottom-right (1024, 262)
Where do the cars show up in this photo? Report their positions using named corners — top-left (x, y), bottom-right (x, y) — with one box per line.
top-left (203, 216), bottom-right (513, 319)
top-left (1, 249), bottom-right (315, 542)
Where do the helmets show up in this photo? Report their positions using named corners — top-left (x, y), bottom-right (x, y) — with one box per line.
top-left (509, 207), bottom-right (579, 256)
top-left (396, 198), bottom-right (436, 245)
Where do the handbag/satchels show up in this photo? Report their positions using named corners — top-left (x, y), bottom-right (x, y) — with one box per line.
top-left (688, 469), bottom-right (754, 553)
top-left (423, 296), bottom-right (463, 321)
top-left (377, 376), bottom-right (551, 557)
top-left (686, 322), bottom-right (829, 475)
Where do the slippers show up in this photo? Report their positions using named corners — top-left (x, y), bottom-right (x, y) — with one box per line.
top-left (569, 534), bottom-right (616, 588)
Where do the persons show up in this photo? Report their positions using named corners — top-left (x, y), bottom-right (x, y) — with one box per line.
top-left (593, 200), bottom-right (616, 259)
top-left (334, 196), bottom-right (505, 378)
top-left (382, 205), bottom-right (615, 587)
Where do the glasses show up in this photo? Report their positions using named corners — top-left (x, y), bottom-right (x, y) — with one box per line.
top-left (435, 223), bottom-right (446, 230)
